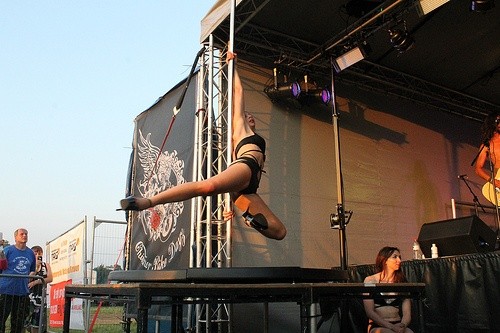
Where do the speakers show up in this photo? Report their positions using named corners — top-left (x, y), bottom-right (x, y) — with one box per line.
top-left (417, 215), bottom-right (498, 258)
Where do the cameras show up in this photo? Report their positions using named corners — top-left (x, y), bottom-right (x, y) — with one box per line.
top-left (38, 256), bottom-right (42, 261)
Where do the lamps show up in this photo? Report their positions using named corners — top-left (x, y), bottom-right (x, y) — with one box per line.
top-left (264, 68), bottom-right (331, 108)
top-left (329, 39), bottom-right (371, 73)
top-left (472, 0), bottom-right (490, 11)
top-left (386, 19), bottom-right (416, 56)
top-left (414, 0), bottom-right (451, 17)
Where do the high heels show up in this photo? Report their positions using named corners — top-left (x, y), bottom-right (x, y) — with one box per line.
top-left (116, 197), bottom-right (152, 212)
top-left (241, 208), bottom-right (269, 231)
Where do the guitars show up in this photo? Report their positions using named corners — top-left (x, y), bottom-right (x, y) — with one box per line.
top-left (482, 166), bottom-right (500, 207)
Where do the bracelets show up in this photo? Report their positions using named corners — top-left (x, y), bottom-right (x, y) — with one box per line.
top-left (489, 177), bottom-right (492, 182)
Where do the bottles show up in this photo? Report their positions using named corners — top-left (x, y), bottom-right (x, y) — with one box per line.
top-left (430, 244), bottom-right (439, 258)
top-left (412, 239), bottom-right (421, 259)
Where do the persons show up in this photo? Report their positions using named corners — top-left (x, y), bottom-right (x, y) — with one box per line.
top-left (475, 114), bottom-right (500, 189)
top-left (0, 227), bottom-right (36, 333)
top-left (362, 246), bottom-right (414, 333)
top-left (0, 240), bottom-right (7, 270)
top-left (24, 246), bottom-right (53, 333)
top-left (116, 52), bottom-right (287, 240)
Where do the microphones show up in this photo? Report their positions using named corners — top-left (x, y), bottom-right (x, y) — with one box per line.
top-left (458, 174), bottom-right (466, 178)
